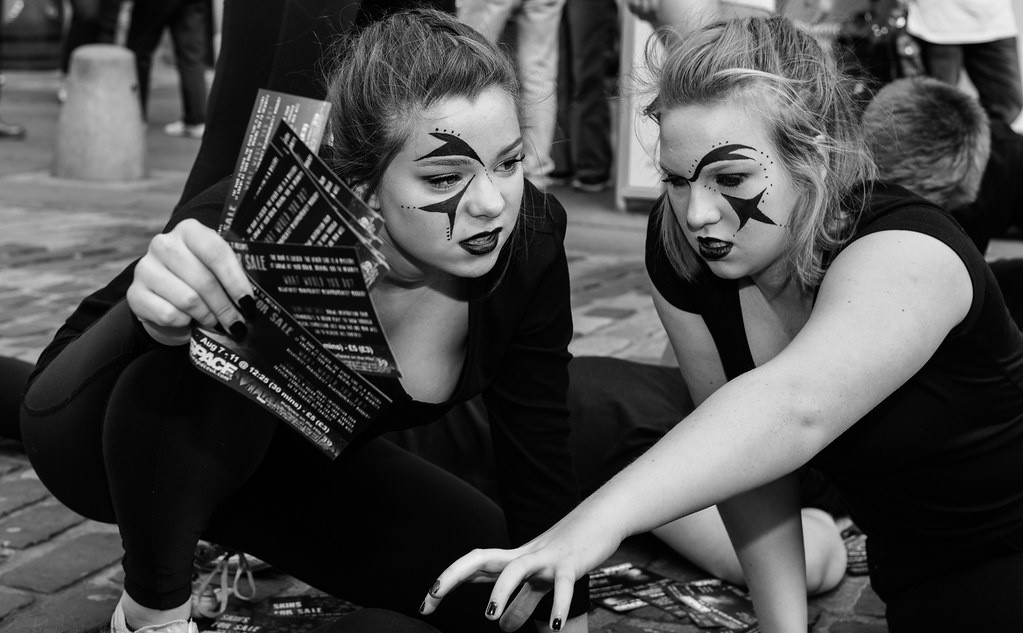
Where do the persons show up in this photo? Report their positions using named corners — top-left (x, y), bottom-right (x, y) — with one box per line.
top-left (19, 0), bottom-right (1023, 633)
top-left (418, 14), bottom-right (1023, 633)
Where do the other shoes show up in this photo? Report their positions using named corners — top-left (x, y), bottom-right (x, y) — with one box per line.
top-left (164, 120), bottom-right (204, 137)
top-left (111, 596), bottom-right (199, 633)
top-left (573, 173), bottom-right (614, 193)
